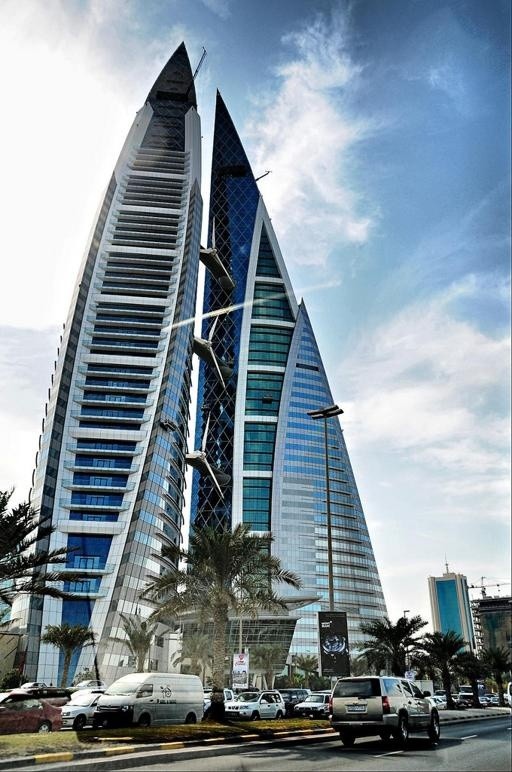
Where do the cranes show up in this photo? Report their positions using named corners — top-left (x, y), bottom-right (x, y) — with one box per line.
top-left (468, 575), bottom-right (512, 596)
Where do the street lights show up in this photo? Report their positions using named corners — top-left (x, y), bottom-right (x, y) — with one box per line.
top-left (403, 609), bottom-right (413, 667)
top-left (308, 401), bottom-right (348, 611)
